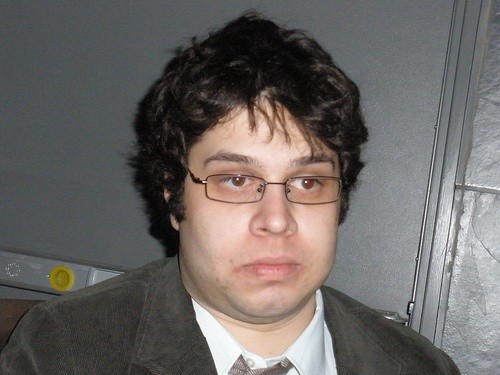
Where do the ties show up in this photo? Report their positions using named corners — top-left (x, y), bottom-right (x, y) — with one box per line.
top-left (230, 354), bottom-right (293, 375)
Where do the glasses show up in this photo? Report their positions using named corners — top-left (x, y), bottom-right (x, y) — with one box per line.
top-left (177, 155), bottom-right (344, 207)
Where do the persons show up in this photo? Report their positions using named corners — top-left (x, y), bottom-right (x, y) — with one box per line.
top-left (2, 11), bottom-right (460, 374)
